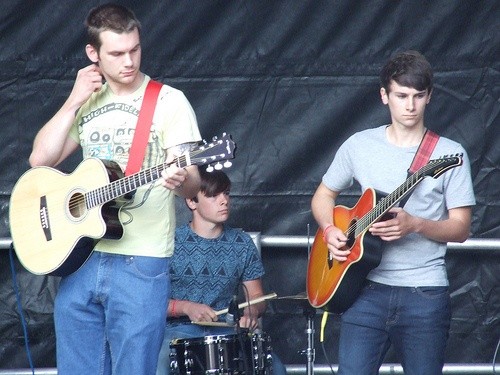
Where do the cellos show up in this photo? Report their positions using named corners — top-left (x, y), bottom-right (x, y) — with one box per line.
top-left (304, 154), bottom-right (463, 312)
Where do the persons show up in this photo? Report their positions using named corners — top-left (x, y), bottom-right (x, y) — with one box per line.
top-left (156, 165), bottom-right (287, 375)
top-left (28, 4), bottom-right (202, 375)
top-left (311, 50), bottom-right (477, 375)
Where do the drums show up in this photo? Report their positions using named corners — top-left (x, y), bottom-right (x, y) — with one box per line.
top-left (170, 332), bottom-right (272, 375)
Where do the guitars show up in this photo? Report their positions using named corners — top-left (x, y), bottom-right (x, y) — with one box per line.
top-left (9, 126), bottom-right (236, 276)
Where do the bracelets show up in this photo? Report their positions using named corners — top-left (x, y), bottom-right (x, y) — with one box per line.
top-left (172, 300), bottom-right (178, 318)
top-left (322, 225), bottom-right (334, 244)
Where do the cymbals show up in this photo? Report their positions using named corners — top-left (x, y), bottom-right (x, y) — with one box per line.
top-left (277, 289), bottom-right (308, 301)
